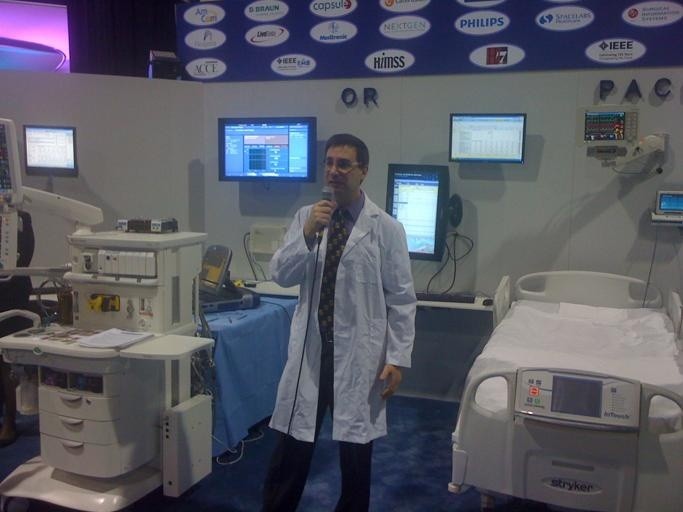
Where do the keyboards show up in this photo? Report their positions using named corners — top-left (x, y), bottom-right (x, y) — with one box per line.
top-left (416, 293), bottom-right (474, 304)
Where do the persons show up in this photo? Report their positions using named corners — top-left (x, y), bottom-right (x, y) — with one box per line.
top-left (261, 133), bottom-right (418, 512)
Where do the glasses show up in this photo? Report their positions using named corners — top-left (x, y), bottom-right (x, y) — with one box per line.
top-left (320, 160), bottom-right (360, 169)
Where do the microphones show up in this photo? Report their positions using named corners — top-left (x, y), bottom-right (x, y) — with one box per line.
top-left (318, 186), bottom-right (335, 244)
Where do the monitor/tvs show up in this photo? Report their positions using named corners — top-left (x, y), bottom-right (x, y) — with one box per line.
top-left (218, 118), bottom-right (317, 181)
top-left (655, 191), bottom-right (683, 215)
top-left (386, 164), bottom-right (449, 261)
top-left (580, 106), bottom-right (637, 146)
top-left (449, 113), bottom-right (527, 163)
top-left (23, 125), bottom-right (78, 176)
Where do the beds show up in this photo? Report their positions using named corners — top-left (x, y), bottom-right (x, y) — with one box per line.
top-left (198, 282), bottom-right (300, 461)
top-left (448, 268), bottom-right (683, 512)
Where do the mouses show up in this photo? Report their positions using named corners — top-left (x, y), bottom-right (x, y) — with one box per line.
top-left (483, 299), bottom-right (493, 305)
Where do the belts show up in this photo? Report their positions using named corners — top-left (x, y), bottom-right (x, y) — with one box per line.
top-left (320, 331), bottom-right (333, 343)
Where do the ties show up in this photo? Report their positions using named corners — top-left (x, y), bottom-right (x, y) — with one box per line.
top-left (318, 211), bottom-right (349, 352)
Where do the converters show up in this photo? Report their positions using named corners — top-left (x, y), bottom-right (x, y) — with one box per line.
top-left (249, 223), bottom-right (288, 256)
